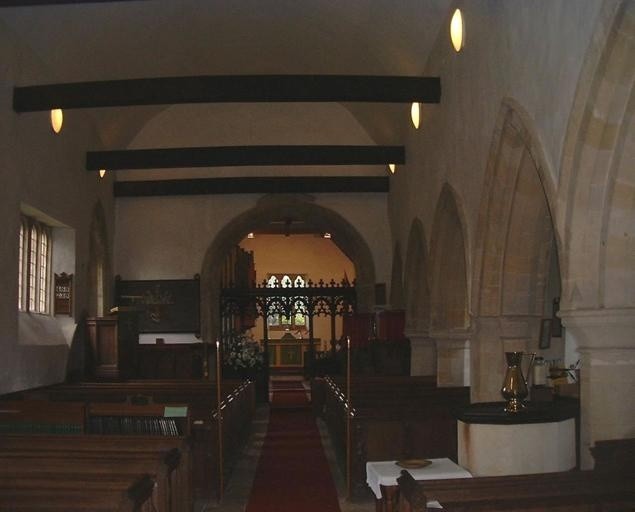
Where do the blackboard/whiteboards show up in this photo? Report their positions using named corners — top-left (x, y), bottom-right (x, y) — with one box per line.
top-left (117, 278), bottom-right (200, 333)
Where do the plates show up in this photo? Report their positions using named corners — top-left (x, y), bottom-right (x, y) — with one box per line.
top-left (396, 459), bottom-right (432, 470)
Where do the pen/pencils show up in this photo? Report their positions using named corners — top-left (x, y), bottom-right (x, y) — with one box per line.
top-left (570, 359), bottom-right (582, 370)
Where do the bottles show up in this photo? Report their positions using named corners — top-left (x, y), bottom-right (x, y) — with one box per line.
top-left (532, 357), bottom-right (547, 388)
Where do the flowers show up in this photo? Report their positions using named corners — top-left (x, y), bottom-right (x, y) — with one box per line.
top-left (221, 330), bottom-right (265, 369)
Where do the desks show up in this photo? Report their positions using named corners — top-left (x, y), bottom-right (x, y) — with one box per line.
top-left (367, 457), bottom-right (472, 512)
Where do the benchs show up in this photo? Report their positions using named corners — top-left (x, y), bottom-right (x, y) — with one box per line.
top-left (323, 373), bottom-right (471, 497)
top-left (0, 376), bottom-right (256, 511)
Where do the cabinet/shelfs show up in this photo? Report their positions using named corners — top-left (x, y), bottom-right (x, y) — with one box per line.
top-left (83, 314), bottom-right (140, 382)
